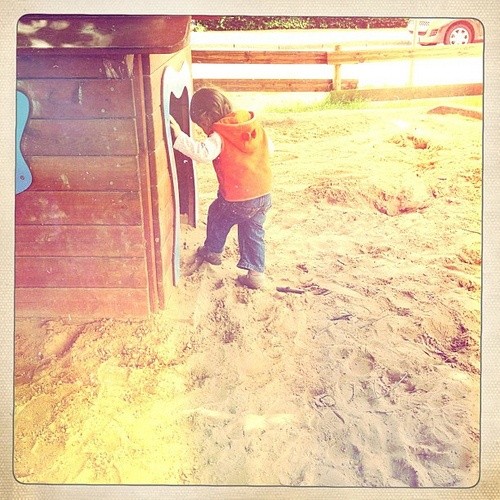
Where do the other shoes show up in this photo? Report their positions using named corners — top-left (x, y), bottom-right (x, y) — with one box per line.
top-left (238, 273), bottom-right (262, 289)
top-left (196, 246), bottom-right (225, 265)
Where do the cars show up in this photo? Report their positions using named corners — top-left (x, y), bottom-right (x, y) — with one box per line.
top-left (407, 18), bottom-right (484, 46)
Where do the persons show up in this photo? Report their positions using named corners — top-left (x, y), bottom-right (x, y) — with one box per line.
top-left (169, 87), bottom-right (274, 289)
